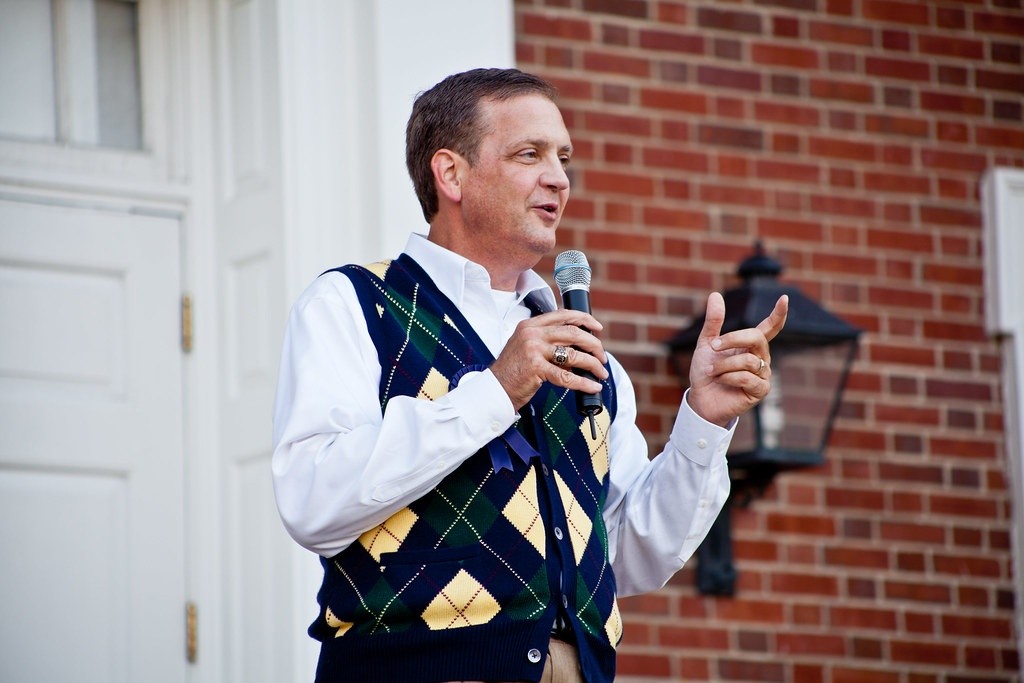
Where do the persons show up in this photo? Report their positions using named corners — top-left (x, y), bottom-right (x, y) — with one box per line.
top-left (270, 67), bottom-right (789, 683)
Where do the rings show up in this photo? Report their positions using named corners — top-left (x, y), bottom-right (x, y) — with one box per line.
top-left (553, 346), bottom-right (568, 366)
top-left (756, 359), bottom-right (766, 375)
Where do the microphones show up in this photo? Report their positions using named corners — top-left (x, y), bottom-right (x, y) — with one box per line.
top-left (554, 249), bottom-right (603, 441)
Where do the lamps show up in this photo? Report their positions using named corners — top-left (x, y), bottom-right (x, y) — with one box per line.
top-left (666, 243), bottom-right (861, 593)
